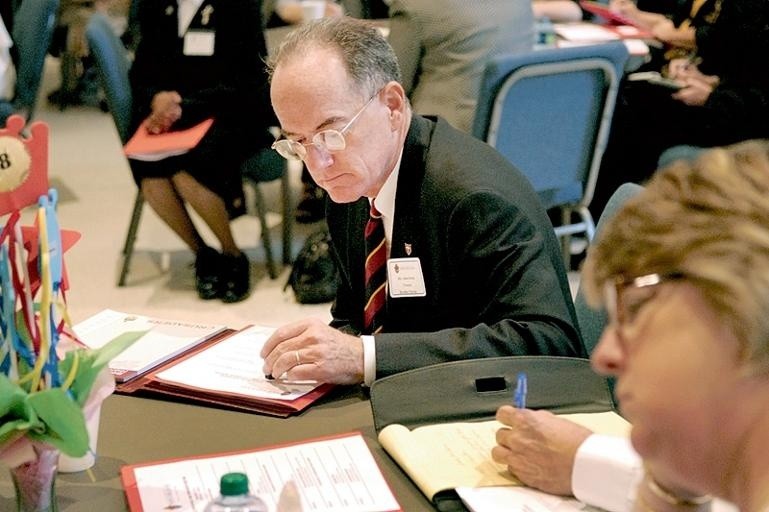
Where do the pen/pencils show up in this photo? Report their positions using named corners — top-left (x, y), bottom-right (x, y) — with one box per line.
top-left (672, 49), bottom-right (699, 81)
top-left (514, 372), bottom-right (527, 409)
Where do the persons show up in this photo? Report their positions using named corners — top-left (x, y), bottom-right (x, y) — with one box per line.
top-left (487, 401), bottom-right (741, 512)
top-left (2, 0), bottom-right (769, 305)
top-left (576, 137), bottom-right (769, 512)
top-left (256, 13), bottom-right (592, 391)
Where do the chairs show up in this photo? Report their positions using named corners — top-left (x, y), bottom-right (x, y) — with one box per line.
top-left (1, 0), bottom-right (57, 120)
top-left (574, 182), bottom-right (650, 405)
top-left (85, 16), bottom-right (294, 290)
top-left (472, 39), bottom-right (630, 277)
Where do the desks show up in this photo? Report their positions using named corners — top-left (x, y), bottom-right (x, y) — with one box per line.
top-left (0, 368), bottom-right (632, 512)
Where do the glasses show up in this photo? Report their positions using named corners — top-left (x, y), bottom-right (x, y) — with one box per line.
top-left (600, 268), bottom-right (688, 328)
top-left (269, 89), bottom-right (380, 164)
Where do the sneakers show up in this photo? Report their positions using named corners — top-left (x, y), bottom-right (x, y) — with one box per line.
top-left (185, 245), bottom-right (258, 307)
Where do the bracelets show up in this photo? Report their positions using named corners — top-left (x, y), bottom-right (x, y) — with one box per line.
top-left (643, 475), bottom-right (714, 508)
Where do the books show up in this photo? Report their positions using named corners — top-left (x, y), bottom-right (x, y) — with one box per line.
top-left (119, 424), bottom-right (406, 512)
top-left (376, 409), bottom-right (636, 512)
top-left (456, 486), bottom-right (604, 512)
top-left (60, 307), bottom-right (346, 420)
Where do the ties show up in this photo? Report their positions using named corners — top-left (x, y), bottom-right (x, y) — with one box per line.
top-left (360, 195), bottom-right (391, 336)
